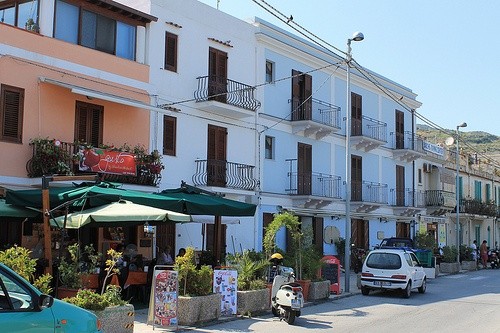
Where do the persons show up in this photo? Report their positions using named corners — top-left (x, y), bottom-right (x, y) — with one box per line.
top-left (480, 240), bottom-right (488, 269)
top-left (470, 240), bottom-right (477, 261)
top-left (157, 245), bottom-right (173, 269)
top-left (117, 244), bottom-right (139, 270)
top-left (179, 248), bottom-right (186, 257)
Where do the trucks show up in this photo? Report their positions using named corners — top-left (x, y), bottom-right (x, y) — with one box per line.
top-left (374, 238), bottom-right (424, 251)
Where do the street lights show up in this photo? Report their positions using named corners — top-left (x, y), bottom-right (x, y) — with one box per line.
top-left (457, 122), bottom-right (467, 263)
top-left (344, 32), bottom-right (364, 293)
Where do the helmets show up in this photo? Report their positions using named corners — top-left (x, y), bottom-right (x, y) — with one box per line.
top-left (269, 253), bottom-right (283, 264)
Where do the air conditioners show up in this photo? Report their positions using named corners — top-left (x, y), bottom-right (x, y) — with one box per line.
top-left (423, 162), bottom-right (432, 174)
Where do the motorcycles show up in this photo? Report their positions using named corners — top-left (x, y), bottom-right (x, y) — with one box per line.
top-left (491, 250), bottom-right (500, 269)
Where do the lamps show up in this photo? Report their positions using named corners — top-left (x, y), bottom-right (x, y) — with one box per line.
top-left (72, 142), bottom-right (100, 177)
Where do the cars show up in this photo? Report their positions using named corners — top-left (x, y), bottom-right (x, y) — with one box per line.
top-left (0, 263), bottom-right (103, 333)
top-left (361, 248), bottom-right (427, 298)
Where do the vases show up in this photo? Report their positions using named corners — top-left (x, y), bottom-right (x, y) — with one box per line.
top-left (150, 166), bottom-right (162, 174)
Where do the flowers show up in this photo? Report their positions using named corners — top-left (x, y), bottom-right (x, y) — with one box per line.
top-left (149, 161), bottom-right (165, 170)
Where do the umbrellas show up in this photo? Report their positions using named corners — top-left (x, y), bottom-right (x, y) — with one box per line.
top-left (50, 199), bottom-right (192, 249)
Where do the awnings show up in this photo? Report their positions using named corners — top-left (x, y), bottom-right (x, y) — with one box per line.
top-left (6, 185), bottom-right (257, 217)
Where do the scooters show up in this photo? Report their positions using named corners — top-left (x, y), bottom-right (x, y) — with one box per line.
top-left (270, 264), bottom-right (304, 325)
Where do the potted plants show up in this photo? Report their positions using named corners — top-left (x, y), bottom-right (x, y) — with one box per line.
top-left (225, 249), bottom-right (284, 315)
top-left (24, 19), bottom-right (40, 33)
top-left (460, 244), bottom-right (476, 270)
top-left (439, 244), bottom-right (459, 273)
top-left (414, 231), bottom-right (439, 276)
top-left (59, 249), bottom-right (135, 333)
top-left (259, 223), bottom-right (331, 302)
top-left (173, 247), bottom-right (222, 326)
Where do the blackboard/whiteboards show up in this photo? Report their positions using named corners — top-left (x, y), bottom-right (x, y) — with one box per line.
top-left (320, 257), bottom-right (340, 293)
